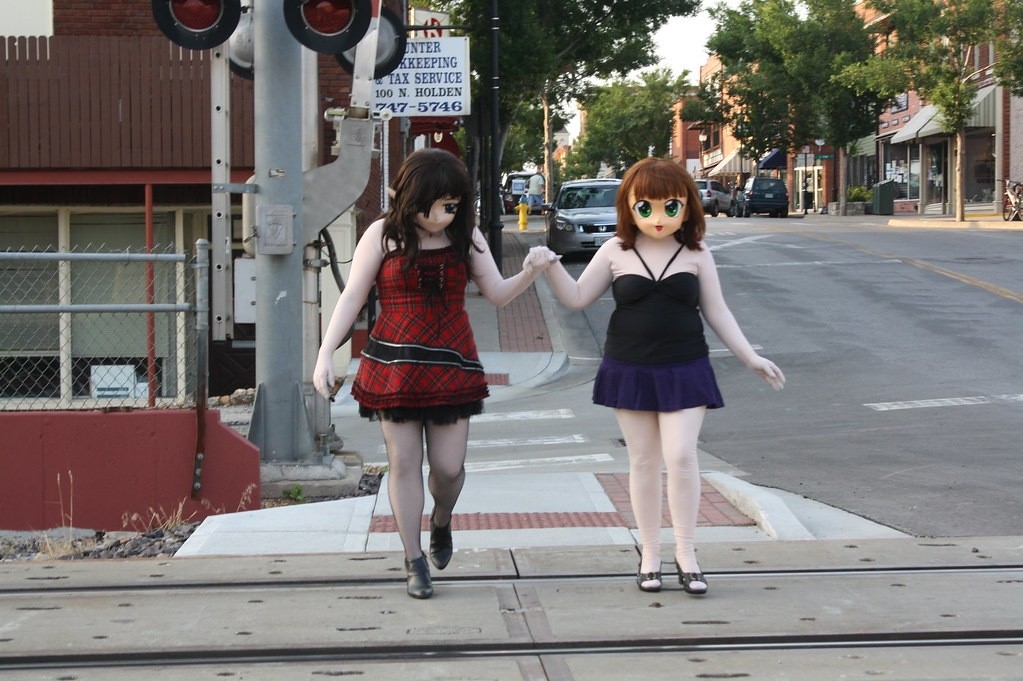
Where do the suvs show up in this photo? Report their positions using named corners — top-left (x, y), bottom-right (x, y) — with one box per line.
top-left (691, 178), bottom-right (736, 216)
top-left (733, 175), bottom-right (789, 218)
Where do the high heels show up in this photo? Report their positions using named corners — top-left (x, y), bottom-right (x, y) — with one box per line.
top-left (636, 553), bottom-right (663, 593)
top-left (675, 556), bottom-right (708, 596)
top-left (428, 503), bottom-right (454, 573)
top-left (404, 550), bottom-right (435, 599)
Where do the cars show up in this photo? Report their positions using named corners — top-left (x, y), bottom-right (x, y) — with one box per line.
top-left (501, 171), bottom-right (546, 215)
top-left (543, 178), bottom-right (633, 258)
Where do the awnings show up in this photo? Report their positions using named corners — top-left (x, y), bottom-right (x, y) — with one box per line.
top-left (707, 147), bottom-right (752, 177)
top-left (759, 147), bottom-right (787, 170)
top-left (890, 84), bottom-right (995, 144)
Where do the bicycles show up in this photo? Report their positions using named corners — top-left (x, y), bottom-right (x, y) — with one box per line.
top-left (995, 178), bottom-right (1023, 222)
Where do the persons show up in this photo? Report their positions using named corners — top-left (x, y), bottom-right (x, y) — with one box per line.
top-left (527, 170), bottom-right (546, 216)
top-left (313, 149), bottom-right (561, 600)
top-left (523, 157), bottom-right (786, 595)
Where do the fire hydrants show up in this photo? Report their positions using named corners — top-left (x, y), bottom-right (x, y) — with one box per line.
top-left (513, 194), bottom-right (531, 229)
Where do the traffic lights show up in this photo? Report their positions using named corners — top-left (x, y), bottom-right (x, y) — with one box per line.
top-left (152, 0), bottom-right (241, 51)
top-left (279, 0), bottom-right (372, 51)
